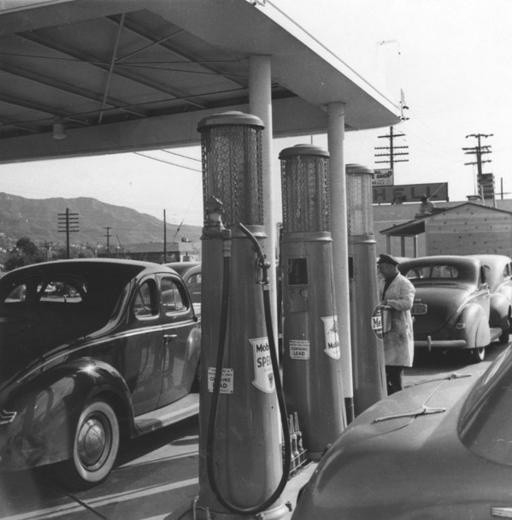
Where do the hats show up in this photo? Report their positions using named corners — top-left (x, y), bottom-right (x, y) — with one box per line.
top-left (376, 254), bottom-right (399, 266)
top-left (419, 194), bottom-right (430, 201)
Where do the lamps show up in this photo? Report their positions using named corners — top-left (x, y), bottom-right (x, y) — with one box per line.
top-left (55, 123), bottom-right (67, 139)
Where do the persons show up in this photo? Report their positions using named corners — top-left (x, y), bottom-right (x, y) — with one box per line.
top-left (376, 253), bottom-right (417, 394)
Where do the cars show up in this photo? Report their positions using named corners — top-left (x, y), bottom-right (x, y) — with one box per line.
top-left (375, 254), bottom-right (512, 364)
top-left (291, 348), bottom-right (512, 519)
